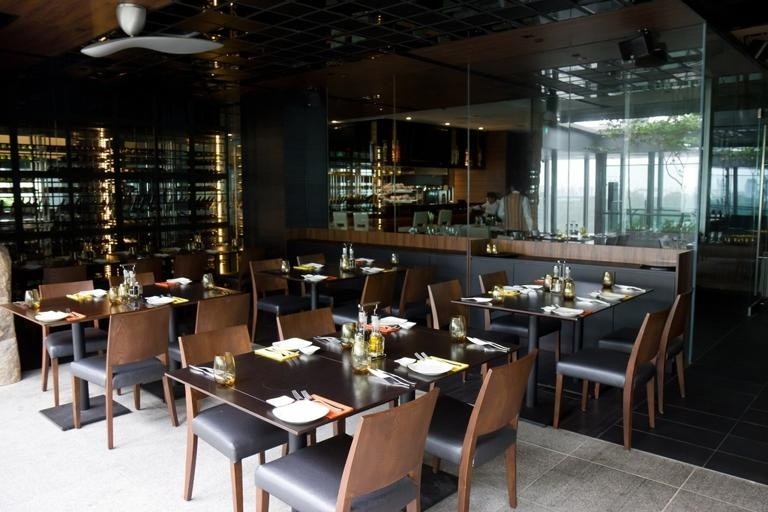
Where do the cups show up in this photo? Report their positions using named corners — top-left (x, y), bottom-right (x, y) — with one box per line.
top-left (601, 270), bottom-right (616, 288)
top-left (25, 289), bottom-right (40, 309)
top-left (214, 351), bottom-right (235, 385)
top-left (391, 250), bottom-right (400, 265)
top-left (202, 273), bottom-right (215, 290)
top-left (450, 315), bottom-right (466, 343)
top-left (281, 261), bottom-right (291, 272)
top-left (409, 224), bottom-right (460, 237)
top-left (491, 283), bottom-right (504, 305)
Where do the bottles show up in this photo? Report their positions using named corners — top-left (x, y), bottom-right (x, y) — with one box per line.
top-left (512, 223), bottom-right (578, 240)
top-left (486, 242), bottom-right (498, 255)
top-left (710, 210), bottom-right (724, 242)
top-left (475, 215), bottom-right (497, 226)
top-left (9, 197), bottom-right (215, 217)
top-left (340, 303), bottom-right (386, 372)
top-left (81, 242), bottom-right (94, 263)
top-left (329, 193), bottom-right (373, 211)
top-left (340, 243), bottom-right (356, 271)
top-left (0, 240), bottom-right (36, 265)
top-left (108, 263), bottom-right (143, 303)
top-left (544, 260), bottom-right (576, 301)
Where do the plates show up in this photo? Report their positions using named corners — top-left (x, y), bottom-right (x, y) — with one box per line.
top-left (355, 257), bottom-right (385, 273)
top-left (300, 262), bottom-right (329, 282)
top-left (35, 310), bottom-right (69, 323)
top-left (272, 401), bottom-right (329, 424)
top-left (145, 276), bottom-right (192, 305)
top-left (553, 307), bottom-right (584, 317)
top-left (380, 316), bottom-right (409, 325)
top-left (79, 288), bottom-right (107, 298)
top-left (474, 297), bottom-right (493, 303)
top-left (503, 285), bottom-right (543, 294)
top-left (597, 291), bottom-right (625, 300)
top-left (407, 362), bottom-right (453, 375)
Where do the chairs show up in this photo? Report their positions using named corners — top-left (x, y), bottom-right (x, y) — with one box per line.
top-left (424, 349), bottom-right (538, 510)
top-left (178, 325), bottom-right (290, 511)
top-left (0, 244), bottom-right (521, 448)
top-left (332, 208), bottom-right (452, 235)
top-left (254, 388), bottom-right (440, 511)
top-left (477, 270), bottom-right (561, 371)
top-left (554, 305), bottom-right (670, 450)
top-left (595, 290), bottom-right (693, 415)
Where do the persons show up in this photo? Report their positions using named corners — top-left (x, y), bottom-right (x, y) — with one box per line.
top-left (498, 181), bottom-right (533, 238)
top-left (470, 192), bottom-right (501, 221)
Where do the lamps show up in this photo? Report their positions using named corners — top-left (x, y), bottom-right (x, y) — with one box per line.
top-left (80, 0), bottom-right (219, 58)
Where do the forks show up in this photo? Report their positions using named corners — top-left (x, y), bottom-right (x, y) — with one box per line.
top-left (265, 347), bottom-right (292, 358)
top-left (413, 351), bottom-right (463, 367)
top-left (314, 336), bottom-right (343, 343)
top-left (292, 389), bottom-right (343, 412)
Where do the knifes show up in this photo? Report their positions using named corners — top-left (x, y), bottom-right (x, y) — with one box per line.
top-left (467, 337), bottom-right (510, 353)
top-left (366, 367), bottom-right (417, 387)
top-left (576, 296), bottom-right (611, 306)
top-left (615, 283), bottom-right (647, 293)
top-left (188, 364), bottom-right (224, 380)
